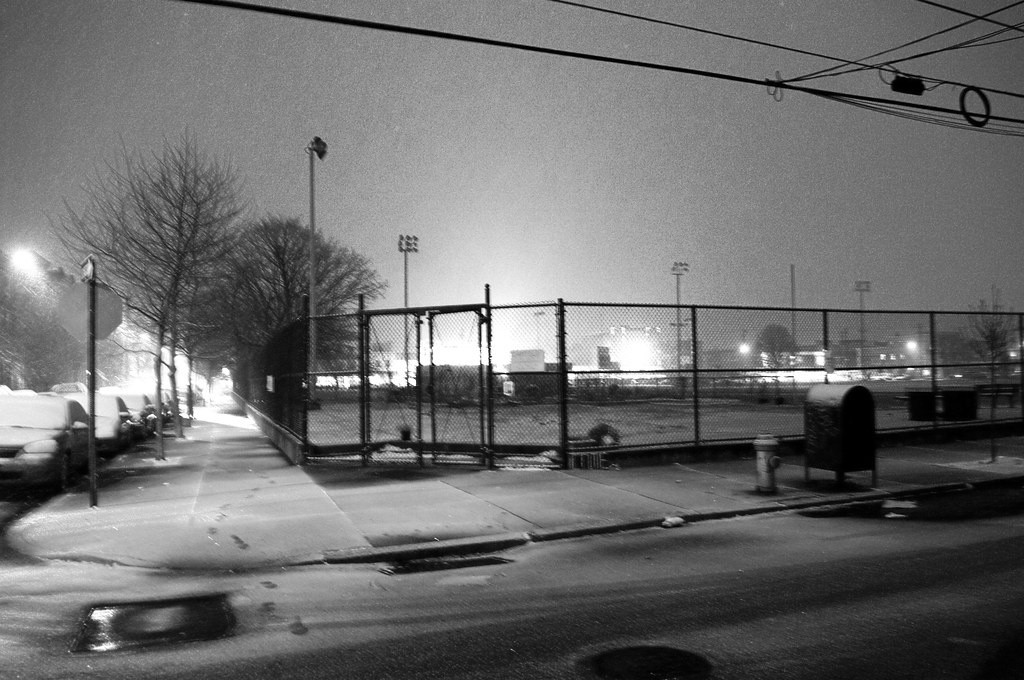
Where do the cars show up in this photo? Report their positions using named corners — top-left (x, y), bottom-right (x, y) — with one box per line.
top-left (0, 378), bottom-right (171, 502)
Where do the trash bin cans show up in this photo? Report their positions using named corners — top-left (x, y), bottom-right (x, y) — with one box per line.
top-left (804, 384), bottom-right (875, 491)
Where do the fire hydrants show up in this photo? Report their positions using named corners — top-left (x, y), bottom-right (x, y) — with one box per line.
top-left (753, 430), bottom-right (782, 492)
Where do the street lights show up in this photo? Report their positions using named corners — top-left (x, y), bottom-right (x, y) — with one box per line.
top-left (396, 232), bottom-right (419, 392)
top-left (854, 279), bottom-right (871, 367)
top-left (12, 247), bottom-right (101, 508)
top-left (670, 261), bottom-right (690, 375)
top-left (303, 135), bottom-right (330, 402)
